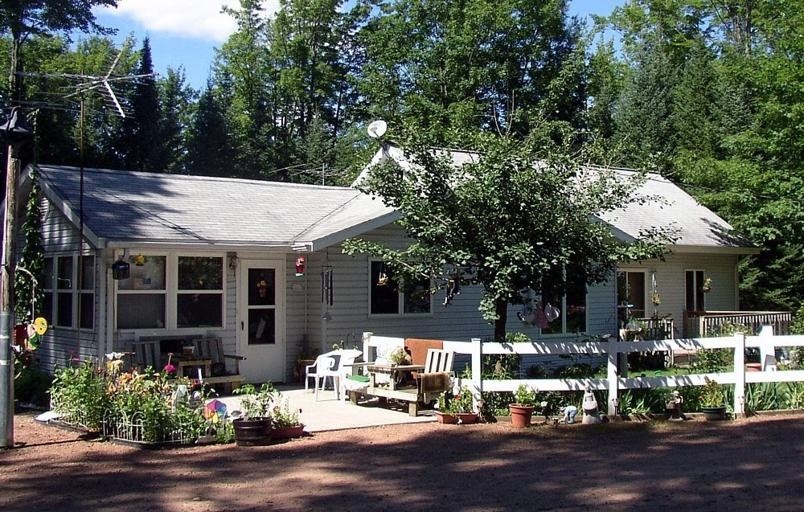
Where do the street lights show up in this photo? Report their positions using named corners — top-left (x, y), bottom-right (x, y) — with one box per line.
top-left (0, 102), bottom-right (32, 446)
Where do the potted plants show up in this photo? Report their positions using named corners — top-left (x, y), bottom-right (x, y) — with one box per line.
top-left (232, 382), bottom-right (305, 446)
top-left (697, 379), bottom-right (728, 420)
top-left (508, 385), bottom-right (540, 427)
top-left (433, 388), bottom-right (478, 424)
top-left (701, 278), bottom-right (713, 294)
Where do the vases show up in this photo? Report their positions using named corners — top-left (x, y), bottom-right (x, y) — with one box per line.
top-left (619, 328), bottom-right (636, 342)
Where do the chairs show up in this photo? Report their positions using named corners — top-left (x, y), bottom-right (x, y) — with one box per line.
top-left (304, 347), bottom-right (362, 402)
top-left (342, 348), bottom-right (454, 418)
top-left (121, 336), bottom-right (246, 397)
